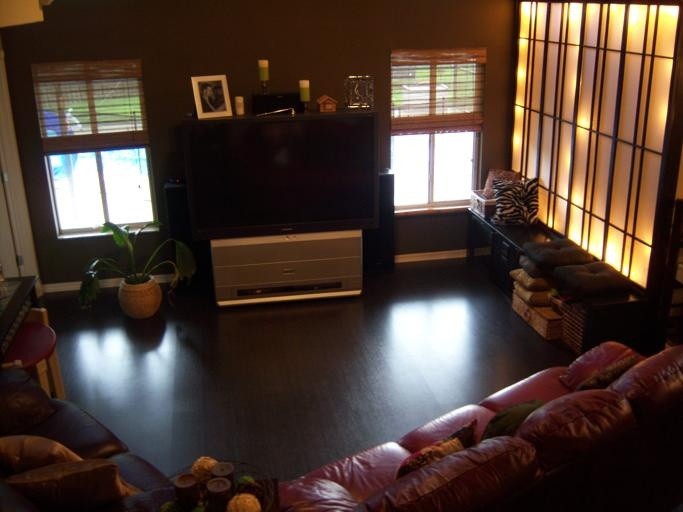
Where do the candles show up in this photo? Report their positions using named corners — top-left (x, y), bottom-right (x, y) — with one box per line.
top-left (259, 60), bottom-right (268, 80)
top-left (299, 80), bottom-right (310, 101)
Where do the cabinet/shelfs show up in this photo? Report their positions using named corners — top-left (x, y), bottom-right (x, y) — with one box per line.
top-left (210, 229), bottom-right (364, 307)
top-left (465, 207), bottom-right (552, 276)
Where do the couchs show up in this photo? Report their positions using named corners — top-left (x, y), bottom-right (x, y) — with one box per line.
top-left (277, 339), bottom-right (683, 512)
top-left (0, 399), bottom-right (177, 511)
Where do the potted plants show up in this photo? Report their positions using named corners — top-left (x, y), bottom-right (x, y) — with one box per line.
top-left (76, 220), bottom-right (192, 319)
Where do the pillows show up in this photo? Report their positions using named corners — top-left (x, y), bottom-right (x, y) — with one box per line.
top-left (0, 436), bottom-right (82, 467)
top-left (575, 357), bottom-right (634, 390)
top-left (395, 438), bottom-right (463, 481)
top-left (483, 168), bottom-right (519, 197)
top-left (489, 178), bottom-right (539, 228)
top-left (480, 399), bottom-right (544, 442)
top-left (0, 462), bottom-right (144, 503)
top-left (509, 238), bottom-right (631, 304)
top-left (0, 382), bottom-right (54, 429)
top-left (442, 419), bottom-right (477, 450)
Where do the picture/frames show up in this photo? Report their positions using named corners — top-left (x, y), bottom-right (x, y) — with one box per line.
top-left (191, 74), bottom-right (232, 118)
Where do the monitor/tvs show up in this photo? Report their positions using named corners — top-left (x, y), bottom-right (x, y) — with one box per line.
top-left (182, 111), bottom-right (380, 241)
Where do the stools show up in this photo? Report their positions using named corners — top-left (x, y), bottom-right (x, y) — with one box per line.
top-left (0, 308), bottom-right (69, 400)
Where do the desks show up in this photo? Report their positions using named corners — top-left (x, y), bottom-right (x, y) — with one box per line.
top-left (164, 459), bottom-right (275, 511)
top-left (0, 275), bottom-right (41, 362)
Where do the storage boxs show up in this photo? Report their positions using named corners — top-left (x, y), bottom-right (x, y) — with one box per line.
top-left (511, 292), bottom-right (561, 339)
top-left (470, 189), bottom-right (498, 221)
